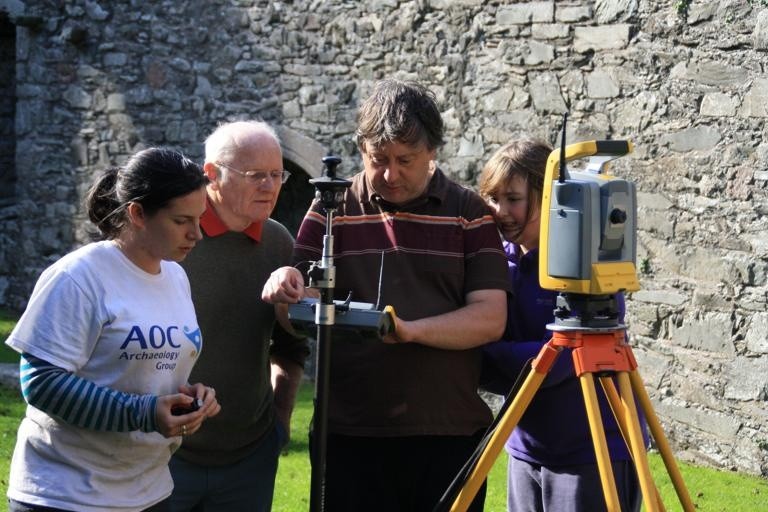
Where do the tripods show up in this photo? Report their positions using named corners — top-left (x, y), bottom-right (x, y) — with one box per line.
top-left (433, 323), bottom-right (695, 512)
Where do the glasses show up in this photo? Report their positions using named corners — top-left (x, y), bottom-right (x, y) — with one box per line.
top-left (215, 161), bottom-right (291, 185)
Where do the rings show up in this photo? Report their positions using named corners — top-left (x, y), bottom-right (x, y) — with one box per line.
top-left (183, 432), bottom-right (187, 437)
top-left (182, 423), bottom-right (186, 431)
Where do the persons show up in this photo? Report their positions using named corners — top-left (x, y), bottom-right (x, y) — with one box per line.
top-left (4, 147), bottom-right (221, 511)
top-left (169, 121), bottom-right (313, 512)
top-left (482, 138), bottom-right (652, 511)
top-left (260, 80), bottom-right (515, 512)
